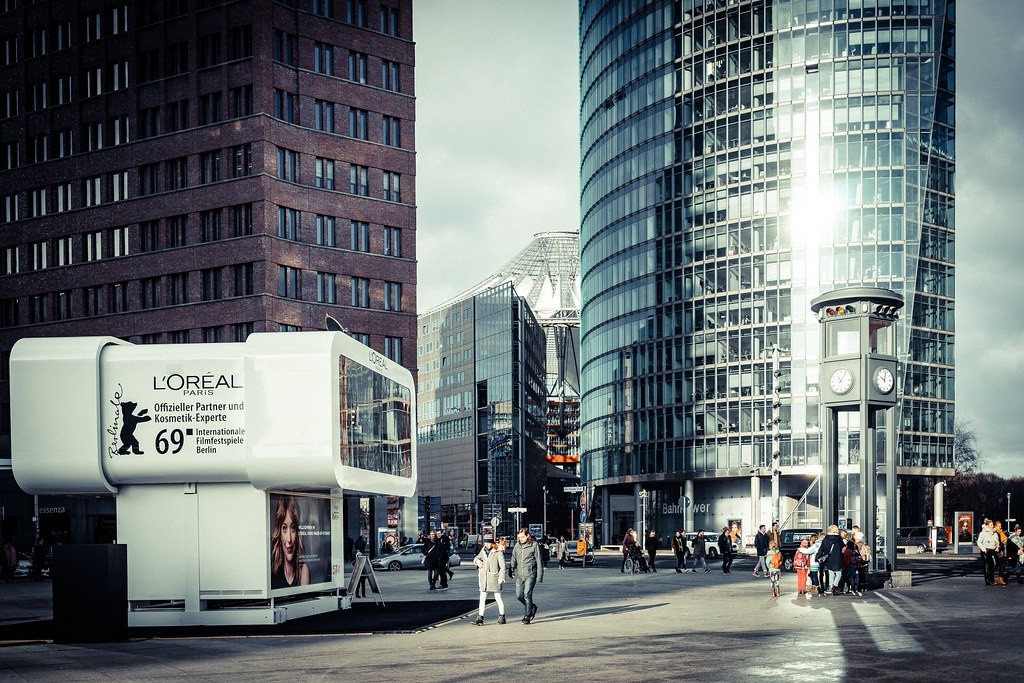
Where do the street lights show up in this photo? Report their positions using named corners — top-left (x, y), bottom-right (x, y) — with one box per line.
top-left (461, 489), bottom-right (473, 535)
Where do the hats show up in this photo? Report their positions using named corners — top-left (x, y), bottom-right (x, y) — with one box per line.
top-left (483, 533), bottom-right (494, 543)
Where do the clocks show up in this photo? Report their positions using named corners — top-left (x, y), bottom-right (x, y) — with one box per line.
top-left (830, 368), bottom-right (854, 395)
top-left (873, 367), bottom-right (895, 395)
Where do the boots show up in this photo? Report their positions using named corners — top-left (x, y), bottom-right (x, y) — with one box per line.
top-left (772, 587), bottom-right (780, 597)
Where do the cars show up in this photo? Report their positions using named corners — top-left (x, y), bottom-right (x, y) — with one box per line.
top-left (500, 536), bottom-right (517, 547)
top-left (564, 541), bottom-right (596, 566)
top-left (547, 537), bottom-right (561, 556)
top-left (370, 544), bottom-right (461, 572)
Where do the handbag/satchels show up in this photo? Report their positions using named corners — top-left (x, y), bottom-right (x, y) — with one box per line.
top-left (421, 555), bottom-right (426, 566)
top-left (818, 554), bottom-right (828, 563)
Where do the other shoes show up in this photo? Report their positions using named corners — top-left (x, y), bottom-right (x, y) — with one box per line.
top-left (449, 572), bottom-right (454, 580)
top-left (363, 594), bottom-right (366, 597)
top-left (991, 581), bottom-right (999, 585)
top-left (435, 586), bottom-right (443, 590)
top-left (800, 584), bottom-right (868, 597)
top-left (752, 571), bottom-right (760, 578)
top-left (430, 585), bottom-right (436, 590)
top-left (653, 569), bottom-right (657, 572)
top-left (496, 615), bottom-right (506, 624)
top-left (521, 615), bottom-right (530, 624)
top-left (723, 570), bottom-right (731, 575)
top-left (997, 577), bottom-right (1007, 585)
top-left (692, 569), bottom-right (696, 573)
top-left (442, 586), bottom-right (449, 591)
top-left (356, 595), bottom-right (361, 598)
top-left (705, 569), bottom-right (711, 573)
top-left (676, 569), bottom-right (682, 574)
top-left (685, 569), bottom-right (689, 572)
top-left (764, 573), bottom-right (770, 577)
top-left (530, 605), bottom-right (538, 620)
top-left (471, 615), bottom-right (483, 626)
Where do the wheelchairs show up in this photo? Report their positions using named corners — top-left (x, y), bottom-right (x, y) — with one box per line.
top-left (625, 546), bottom-right (650, 574)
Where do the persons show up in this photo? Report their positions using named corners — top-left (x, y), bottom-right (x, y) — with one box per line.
top-left (474, 536), bottom-right (569, 571)
top-left (977, 519), bottom-right (1024, 586)
top-left (422, 529), bottom-right (454, 591)
top-left (448, 529), bottom-right (456, 541)
top-left (0, 540), bottom-right (17, 585)
top-left (272, 495), bottom-right (310, 590)
top-left (400, 533), bottom-right (424, 552)
top-left (730, 522), bottom-right (741, 545)
top-left (31, 537), bottom-right (45, 581)
top-left (619, 528), bottom-right (658, 573)
top-left (752, 522), bottom-right (780, 576)
top-left (692, 528), bottom-right (711, 574)
top-left (718, 527), bottom-right (734, 575)
top-left (765, 541), bottom-right (781, 597)
top-left (959, 520), bottom-right (972, 542)
top-left (471, 537), bottom-right (506, 625)
top-left (344, 534), bottom-right (367, 598)
top-left (792, 524), bottom-right (871, 597)
top-left (671, 529), bottom-right (689, 573)
top-left (509, 527), bottom-right (543, 625)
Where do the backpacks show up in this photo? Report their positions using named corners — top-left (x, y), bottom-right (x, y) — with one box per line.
top-left (767, 552), bottom-right (782, 569)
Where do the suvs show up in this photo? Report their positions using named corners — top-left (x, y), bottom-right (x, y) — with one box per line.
top-left (685, 532), bottom-right (739, 559)
top-left (779, 528), bottom-right (845, 571)
top-left (879, 526), bottom-right (949, 554)
